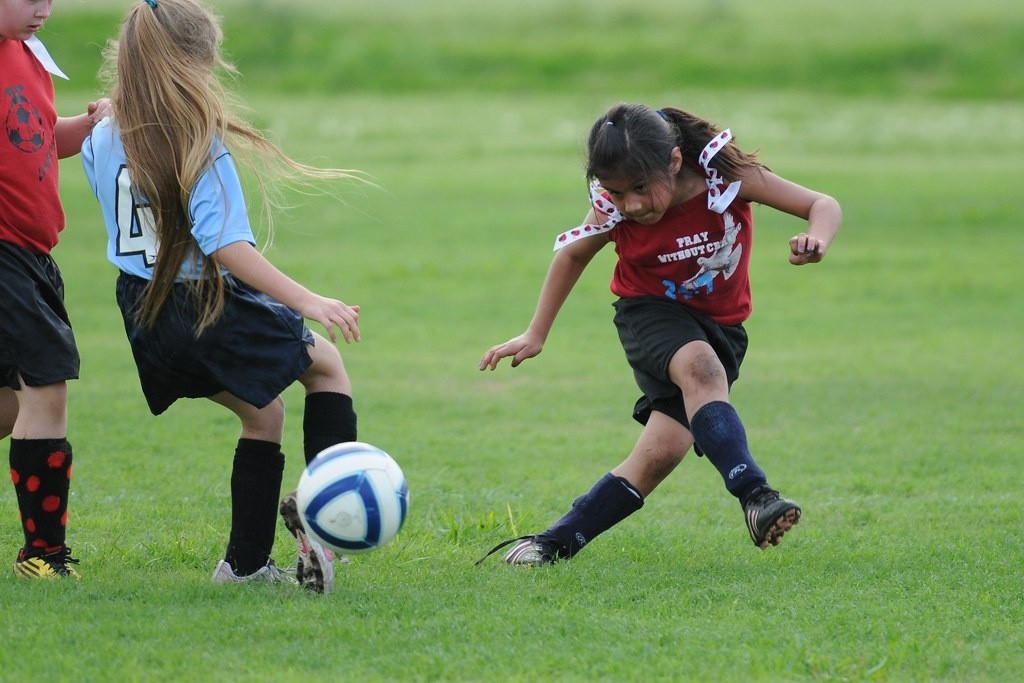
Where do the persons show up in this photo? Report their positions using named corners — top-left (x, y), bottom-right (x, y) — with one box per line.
top-left (0, 0), bottom-right (119, 578)
top-left (80, 0), bottom-right (362, 596)
top-left (478, 104), bottom-right (842, 563)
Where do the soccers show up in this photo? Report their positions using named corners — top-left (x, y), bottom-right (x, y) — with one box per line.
top-left (293, 440), bottom-right (412, 555)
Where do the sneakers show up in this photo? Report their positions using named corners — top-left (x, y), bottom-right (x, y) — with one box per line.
top-left (13, 546), bottom-right (82, 582)
top-left (279, 490), bottom-right (333, 594)
top-left (502, 535), bottom-right (562, 566)
top-left (212, 557), bottom-right (300, 586)
top-left (745, 483), bottom-right (801, 551)
